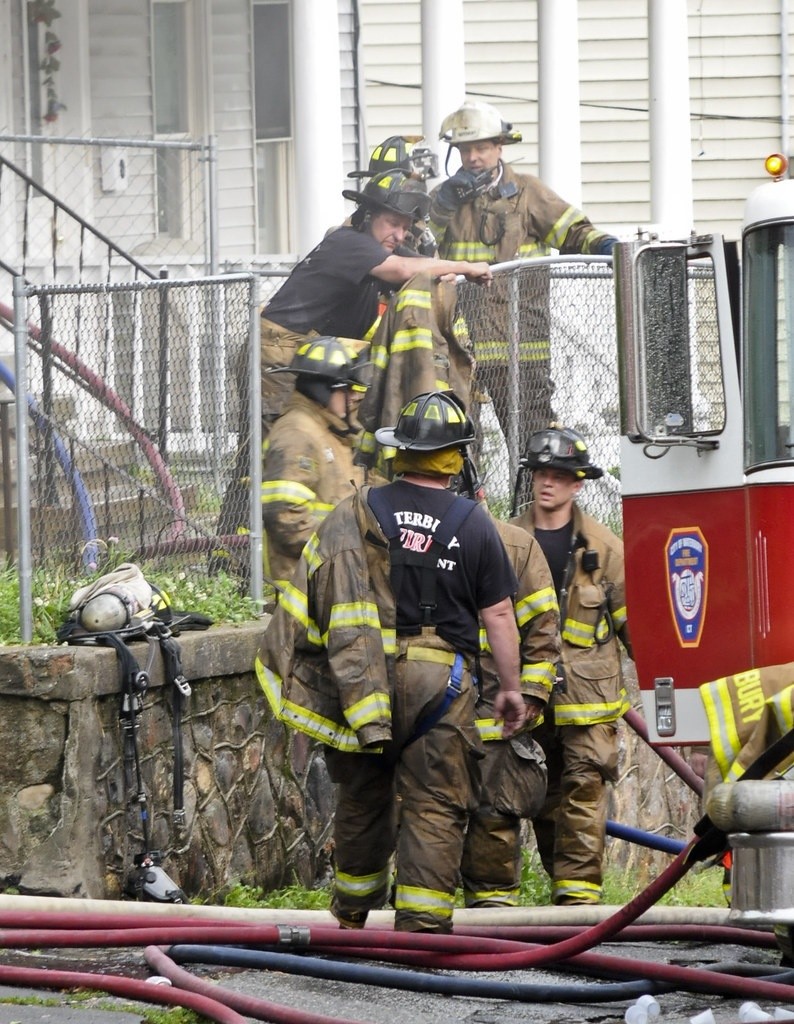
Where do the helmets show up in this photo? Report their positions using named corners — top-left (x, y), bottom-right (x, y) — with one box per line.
top-left (374, 393), bottom-right (475, 450)
top-left (347, 136), bottom-right (439, 182)
top-left (265, 339), bottom-right (354, 381)
top-left (438, 101), bottom-right (521, 145)
top-left (519, 427), bottom-right (603, 479)
top-left (342, 168), bottom-right (428, 222)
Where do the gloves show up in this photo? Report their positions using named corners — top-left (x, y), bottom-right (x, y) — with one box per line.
top-left (598, 237), bottom-right (619, 270)
top-left (436, 173), bottom-right (481, 210)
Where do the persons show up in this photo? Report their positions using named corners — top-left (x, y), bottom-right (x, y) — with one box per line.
top-left (448, 456), bottom-right (562, 908)
top-left (262, 336), bottom-right (391, 607)
top-left (208, 135), bottom-right (493, 578)
top-left (504, 427), bottom-right (714, 906)
top-left (254, 389), bottom-right (528, 935)
top-left (427, 103), bottom-right (623, 516)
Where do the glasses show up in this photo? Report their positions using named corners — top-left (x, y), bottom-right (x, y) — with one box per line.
top-left (393, 154), bottom-right (438, 181)
top-left (389, 192), bottom-right (431, 218)
top-left (346, 362), bottom-right (374, 384)
top-left (527, 430), bottom-right (580, 460)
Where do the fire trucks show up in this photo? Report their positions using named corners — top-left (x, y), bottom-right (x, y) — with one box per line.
top-left (613, 153), bottom-right (794, 747)
